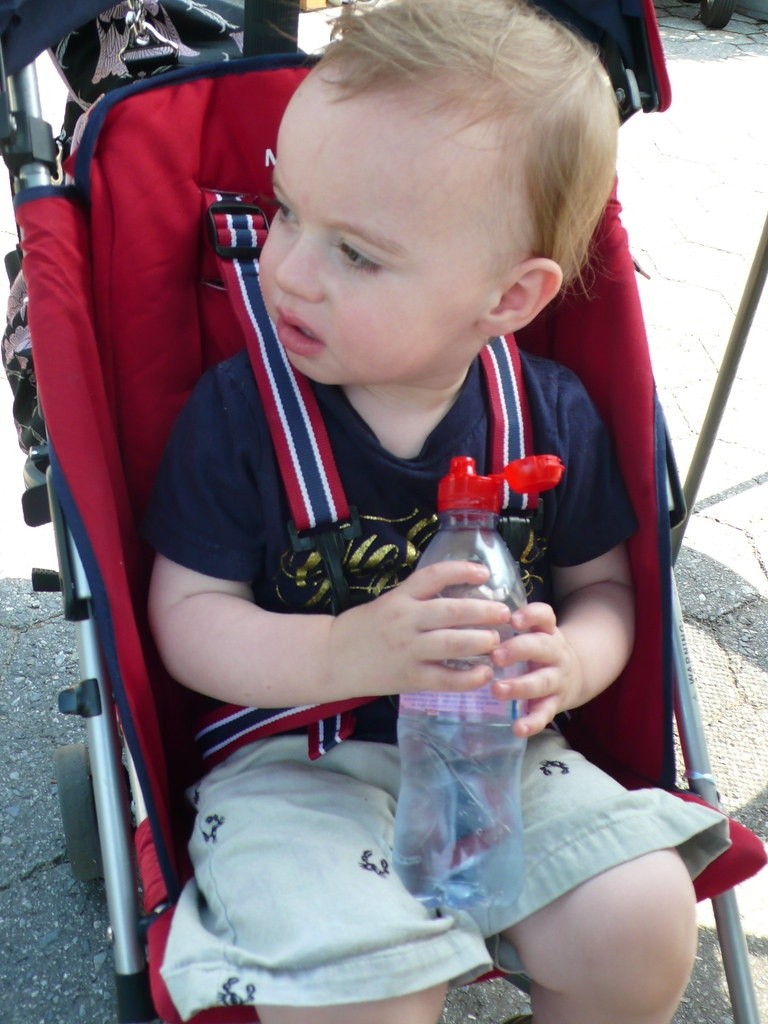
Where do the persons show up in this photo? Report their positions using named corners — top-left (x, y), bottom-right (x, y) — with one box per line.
top-left (148, 1), bottom-right (734, 1024)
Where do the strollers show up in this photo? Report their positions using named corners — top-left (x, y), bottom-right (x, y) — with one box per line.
top-left (2, 0), bottom-right (768, 1022)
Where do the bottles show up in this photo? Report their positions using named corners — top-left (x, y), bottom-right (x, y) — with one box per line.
top-left (391, 455), bottom-right (564, 910)
